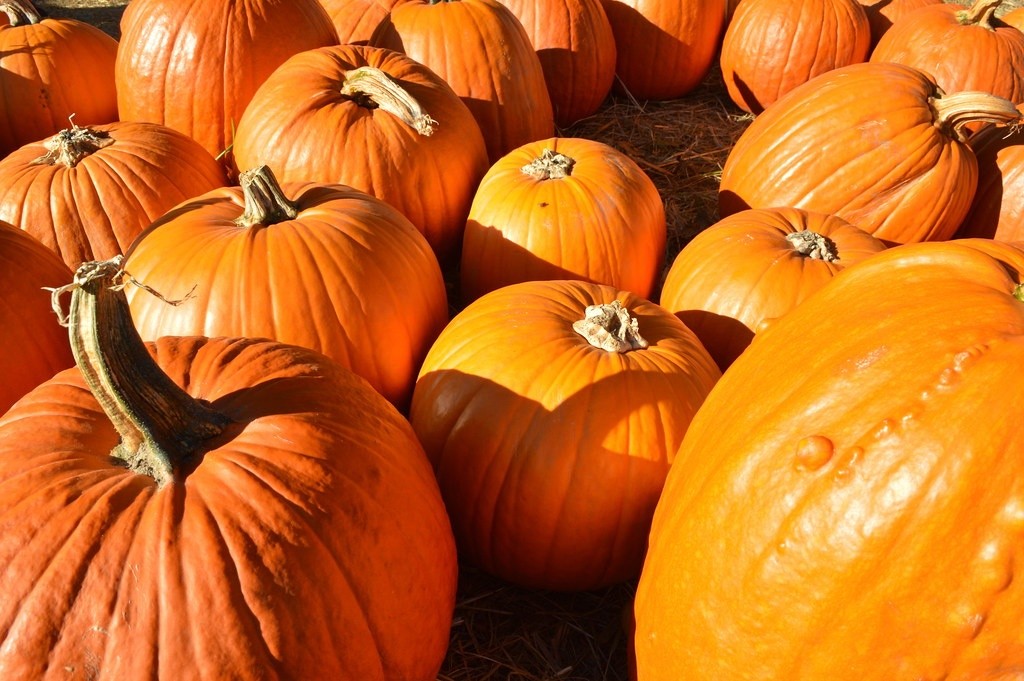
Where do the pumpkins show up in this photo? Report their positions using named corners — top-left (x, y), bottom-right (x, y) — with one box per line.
top-left (0, 1), bottom-right (1024, 679)
